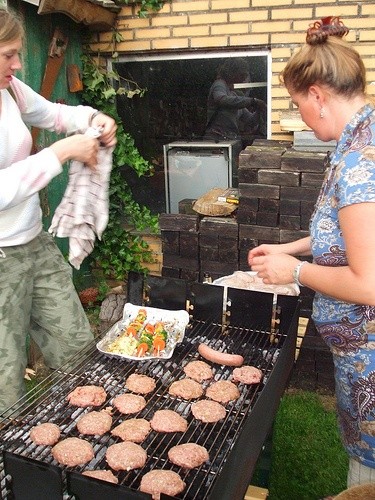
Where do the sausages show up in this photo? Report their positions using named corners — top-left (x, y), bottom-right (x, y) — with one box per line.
top-left (198, 343), bottom-right (244, 366)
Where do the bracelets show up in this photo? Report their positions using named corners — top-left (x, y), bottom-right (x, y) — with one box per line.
top-left (293, 261), bottom-right (307, 287)
top-left (91, 111), bottom-right (100, 122)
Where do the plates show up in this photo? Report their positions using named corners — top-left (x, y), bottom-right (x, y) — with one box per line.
top-left (96, 303), bottom-right (190, 361)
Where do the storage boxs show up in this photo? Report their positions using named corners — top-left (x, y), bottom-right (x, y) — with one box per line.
top-left (129, 229), bottom-right (163, 277)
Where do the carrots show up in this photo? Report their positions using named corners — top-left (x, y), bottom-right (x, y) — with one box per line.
top-left (128, 309), bottom-right (167, 354)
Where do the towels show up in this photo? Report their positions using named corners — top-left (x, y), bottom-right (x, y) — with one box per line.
top-left (48, 126), bottom-right (116, 269)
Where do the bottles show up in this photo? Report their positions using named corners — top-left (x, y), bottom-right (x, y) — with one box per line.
top-left (202, 271), bottom-right (212, 285)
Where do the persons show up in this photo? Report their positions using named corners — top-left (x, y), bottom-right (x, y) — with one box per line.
top-left (249, 16), bottom-right (375, 492)
top-left (204, 58), bottom-right (267, 142)
top-left (0, 7), bottom-right (117, 424)
